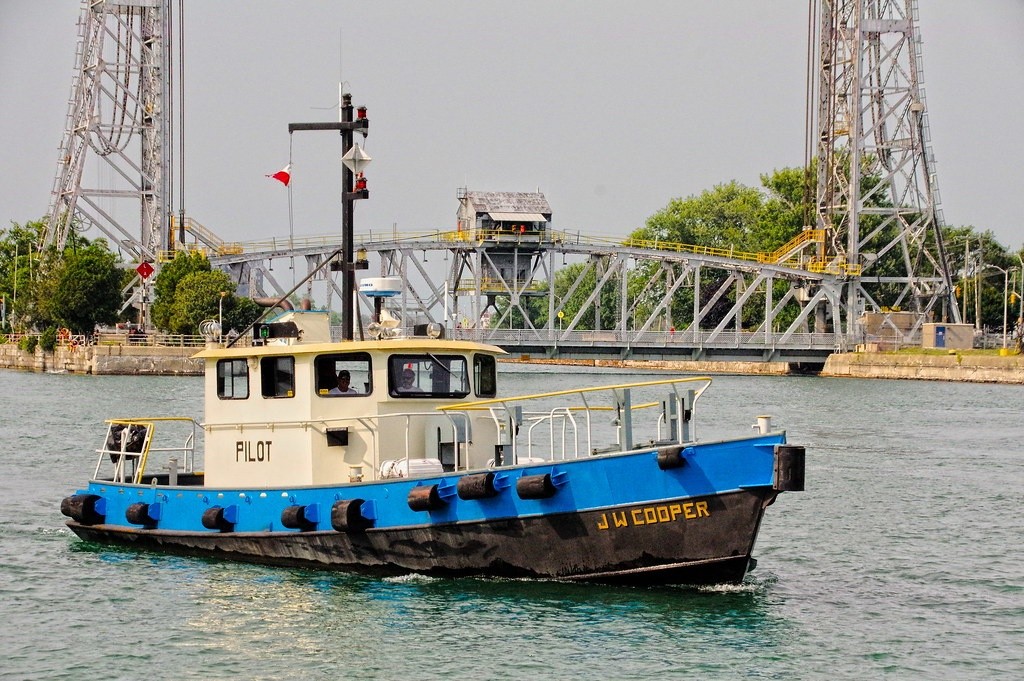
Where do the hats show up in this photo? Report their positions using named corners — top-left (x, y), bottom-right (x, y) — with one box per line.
top-left (339, 370), bottom-right (350, 377)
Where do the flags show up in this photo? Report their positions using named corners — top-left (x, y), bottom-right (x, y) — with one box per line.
top-left (265, 164), bottom-right (292, 188)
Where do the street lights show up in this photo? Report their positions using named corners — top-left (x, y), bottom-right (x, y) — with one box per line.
top-left (985, 264), bottom-right (1009, 348)
top-left (218, 291), bottom-right (227, 343)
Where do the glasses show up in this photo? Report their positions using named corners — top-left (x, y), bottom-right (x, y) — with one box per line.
top-left (401, 376), bottom-right (413, 381)
top-left (339, 376), bottom-right (350, 380)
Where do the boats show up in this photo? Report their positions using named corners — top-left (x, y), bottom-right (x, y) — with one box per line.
top-left (57, 83), bottom-right (808, 593)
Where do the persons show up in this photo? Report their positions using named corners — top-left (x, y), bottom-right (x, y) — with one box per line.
top-left (457, 322), bottom-right (463, 337)
top-left (125, 319), bottom-right (145, 338)
top-left (393, 369), bottom-right (425, 396)
top-left (325, 369), bottom-right (358, 395)
